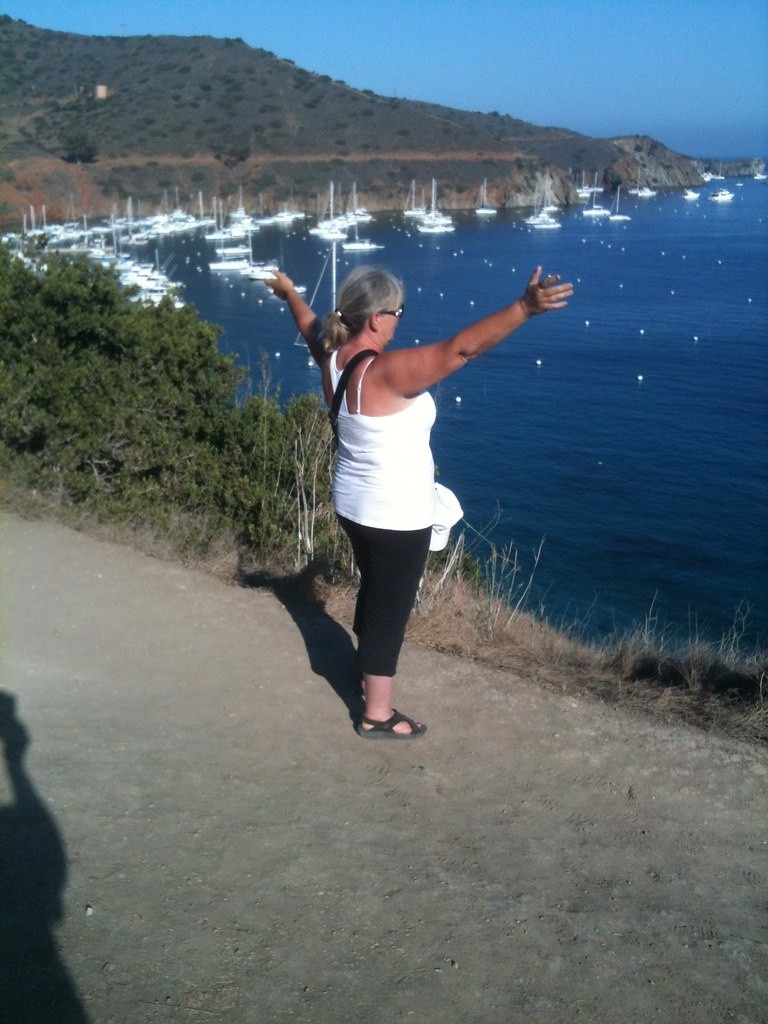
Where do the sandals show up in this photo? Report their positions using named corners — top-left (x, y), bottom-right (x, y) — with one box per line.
top-left (354, 674), bottom-right (364, 701)
top-left (357, 708), bottom-right (427, 740)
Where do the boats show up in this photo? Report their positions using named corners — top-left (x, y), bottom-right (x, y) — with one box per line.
top-left (754, 173), bottom-right (767, 179)
top-left (736, 183), bottom-right (743, 186)
top-left (702, 161), bottom-right (724, 181)
top-left (684, 190), bottom-right (700, 200)
top-left (709, 188), bottom-right (734, 201)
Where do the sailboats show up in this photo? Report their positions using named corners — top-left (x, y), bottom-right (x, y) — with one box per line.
top-left (524, 170), bottom-right (562, 228)
top-left (309, 180), bottom-right (384, 252)
top-left (403, 178), bottom-right (454, 233)
top-left (475, 177), bottom-right (497, 214)
top-left (205, 185), bottom-right (307, 295)
top-left (627, 168), bottom-right (657, 197)
top-left (576, 170), bottom-right (632, 221)
top-left (1, 186), bottom-right (217, 310)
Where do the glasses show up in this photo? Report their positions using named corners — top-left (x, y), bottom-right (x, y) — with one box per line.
top-left (379, 305), bottom-right (404, 320)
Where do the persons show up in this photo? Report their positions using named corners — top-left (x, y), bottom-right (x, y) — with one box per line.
top-left (267, 263), bottom-right (574, 739)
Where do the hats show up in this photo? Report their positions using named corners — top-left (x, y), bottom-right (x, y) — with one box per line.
top-left (429, 483), bottom-right (464, 550)
